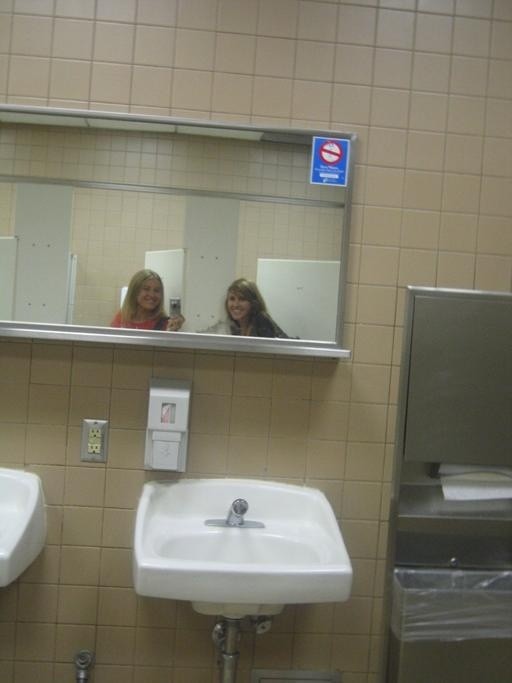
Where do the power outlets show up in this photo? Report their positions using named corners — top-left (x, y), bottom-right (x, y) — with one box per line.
top-left (80, 419), bottom-right (109, 463)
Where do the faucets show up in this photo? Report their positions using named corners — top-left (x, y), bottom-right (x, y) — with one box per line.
top-left (204, 499), bottom-right (265, 528)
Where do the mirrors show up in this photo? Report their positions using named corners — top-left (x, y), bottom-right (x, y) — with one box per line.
top-left (0, 104), bottom-right (359, 360)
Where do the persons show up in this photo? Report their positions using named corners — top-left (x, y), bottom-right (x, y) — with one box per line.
top-left (109, 269), bottom-right (186, 330)
top-left (227, 279), bottom-right (297, 338)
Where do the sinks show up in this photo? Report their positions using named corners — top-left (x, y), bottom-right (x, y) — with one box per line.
top-left (0, 468), bottom-right (46, 587)
top-left (133, 479), bottom-right (352, 618)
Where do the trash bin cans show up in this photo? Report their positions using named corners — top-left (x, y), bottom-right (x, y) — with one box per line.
top-left (387, 569), bottom-right (511, 678)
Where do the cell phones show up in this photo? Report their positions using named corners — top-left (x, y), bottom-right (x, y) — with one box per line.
top-left (169, 298), bottom-right (180, 321)
